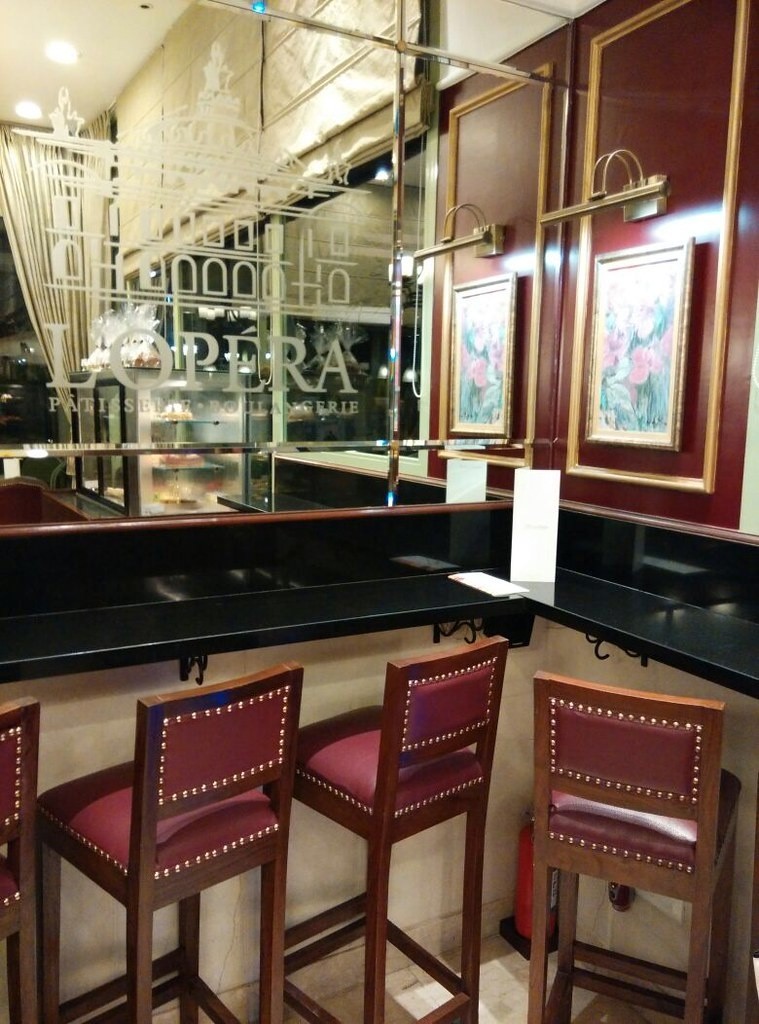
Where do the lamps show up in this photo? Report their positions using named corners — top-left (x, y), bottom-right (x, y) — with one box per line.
top-left (388, 255), bottom-right (424, 308)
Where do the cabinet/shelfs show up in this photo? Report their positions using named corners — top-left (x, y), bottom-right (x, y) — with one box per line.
top-left (68, 366), bottom-right (251, 517)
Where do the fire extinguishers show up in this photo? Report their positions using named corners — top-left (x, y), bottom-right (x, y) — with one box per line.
top-left (518, 813), bottom-right (561, 940)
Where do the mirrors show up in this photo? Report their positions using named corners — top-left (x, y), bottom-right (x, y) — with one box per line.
top-left (0, 1), bottom-right (574, 539)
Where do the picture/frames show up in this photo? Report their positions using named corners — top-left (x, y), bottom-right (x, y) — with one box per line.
top-left (448, 272), bottom-right (517, 438)
top-left (584, 236), bottom-right (697, 452)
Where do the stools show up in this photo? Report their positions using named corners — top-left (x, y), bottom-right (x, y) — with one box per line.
top-left (283, 635), bottom-right (509, 1024)
top-left (526, 671), bottom-right (744, 1023)
top-left (34, 659), bottom-right (305, 1024)
top-left (0, 695), bottom-right (42, 1024)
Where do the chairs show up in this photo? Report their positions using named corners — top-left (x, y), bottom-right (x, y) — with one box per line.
top-left (20, 456), bottom-right (68, 488)
top-left (0, 476), bottom-right (48, 524)
